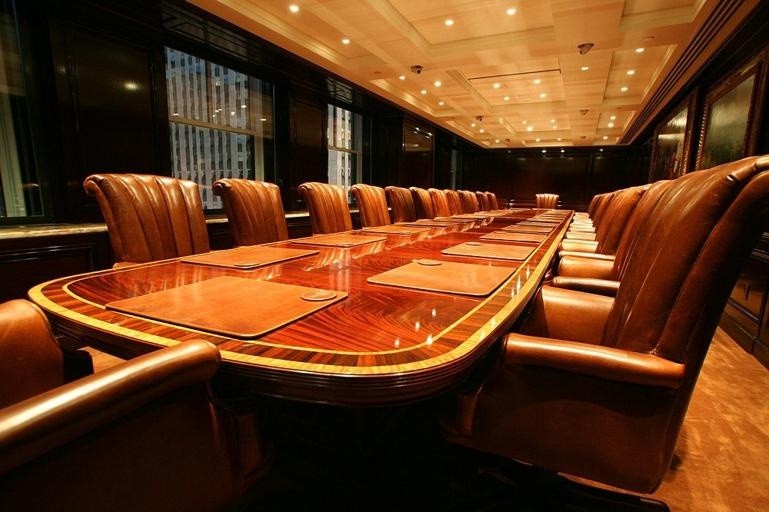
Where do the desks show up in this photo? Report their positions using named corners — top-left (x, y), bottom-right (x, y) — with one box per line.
top-left (30, 206), bottom-right (572, 408)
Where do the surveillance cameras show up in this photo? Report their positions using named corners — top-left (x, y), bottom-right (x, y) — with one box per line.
top-left (579, 45), bottom-right (592, 55)
top-left (411, 65), bottom-right (422, 74)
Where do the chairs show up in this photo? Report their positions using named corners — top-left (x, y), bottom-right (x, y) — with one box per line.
top-left (0, 294), bottom-right (269, 512)
top-left (83, 174), bottom-right (211, 269)
top-left (543, 179), bottom-right (673, 296)
top-left (214, 178), bottom-right (288, 247)
top-left (534, 193), bottom-right (561, 209)
top-left (297, 182), bottom-right (497, 238)
top-left (451, 155), bottom-right (769, 512)
